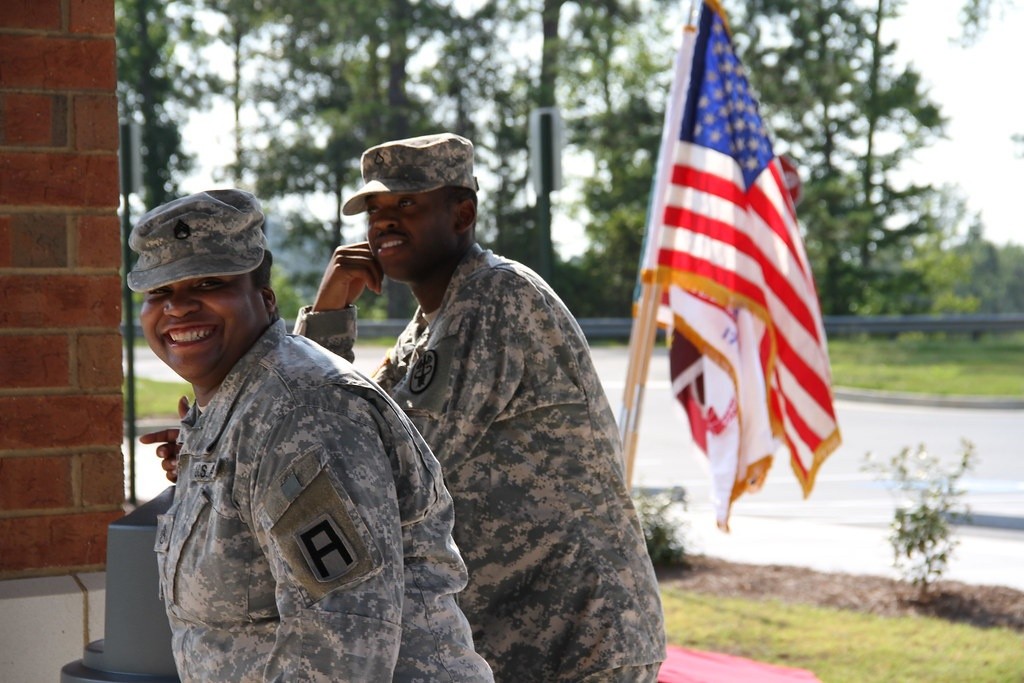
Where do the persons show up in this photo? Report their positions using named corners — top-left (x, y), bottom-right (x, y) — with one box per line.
top-left (140, 132), bottom-right (667, 683)
top-left (127, 188), bottom-right (498, 683)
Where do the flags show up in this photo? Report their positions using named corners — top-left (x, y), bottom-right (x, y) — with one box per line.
top-left (632, 0), bottom-right (841, 533)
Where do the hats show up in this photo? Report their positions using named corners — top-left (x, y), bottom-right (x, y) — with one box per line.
top-left (341, 133), bottom-right (479, 215)
top-left (126, 190), bottom-right (272, 292)
